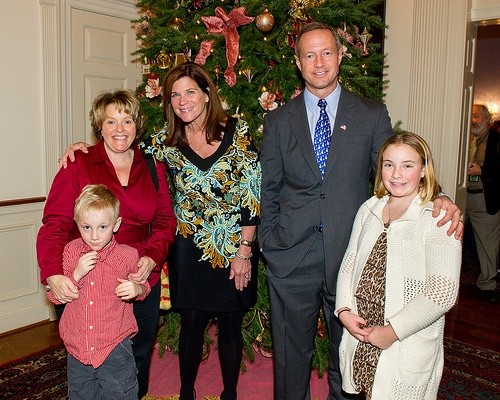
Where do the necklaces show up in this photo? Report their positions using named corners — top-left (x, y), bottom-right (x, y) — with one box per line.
top-left (384, 198), bottom-right (406, 227)
top-left (191, 140), bottom-right (207, 153)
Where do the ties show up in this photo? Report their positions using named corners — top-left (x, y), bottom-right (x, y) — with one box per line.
top-left (313, 99), bottom-right (332, 231)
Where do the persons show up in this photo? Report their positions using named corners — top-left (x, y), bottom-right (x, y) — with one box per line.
top-left (46, 185), bottom-right (151, 400)
top-left (58, 62), bottom-right (263, 400)
top-left (260, 21), bottom-right (464, 400)
top-left (461, 105), bottom-right (500, 301)
top-left (36, 87), bottom-right (178, 400)
top-left (334, 130), bottom-right (462, 400)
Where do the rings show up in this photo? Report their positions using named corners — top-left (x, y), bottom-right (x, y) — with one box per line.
top-left (459, 219), bottom-right (464, 224)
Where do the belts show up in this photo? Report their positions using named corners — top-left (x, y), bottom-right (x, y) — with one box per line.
top-left (466, 189), bottom-right (485, 194)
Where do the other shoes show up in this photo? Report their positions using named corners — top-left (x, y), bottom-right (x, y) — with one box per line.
top-left (479, 290), bottom-right (498, 304)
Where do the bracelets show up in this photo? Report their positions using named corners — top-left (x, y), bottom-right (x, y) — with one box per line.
top-left (240, 239), bottom-right (252, 246)
top-left (236, 251), bottom-right (253, 260)
top-left (338, 310), bottom-right (350, 318)
